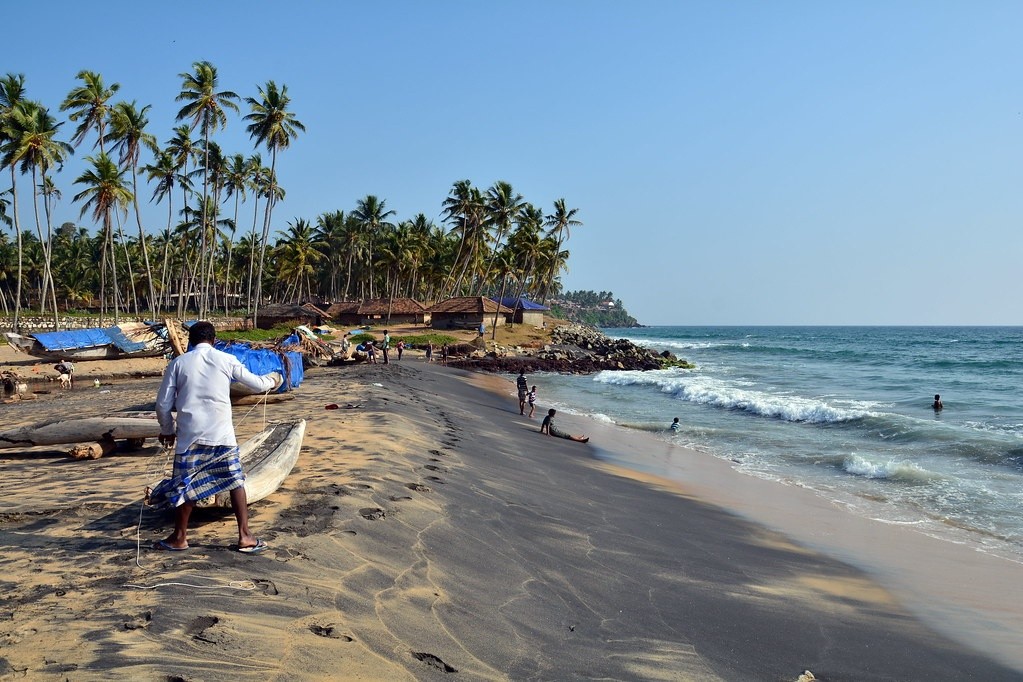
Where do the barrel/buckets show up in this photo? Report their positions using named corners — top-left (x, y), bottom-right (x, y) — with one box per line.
top-left (4, 382), bottom-right (27, 393)
top-left (94, 378), bottom-right (100, 387)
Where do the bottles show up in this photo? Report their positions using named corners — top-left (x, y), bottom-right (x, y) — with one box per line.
top-left (163, 352), bottom-right (172, 360)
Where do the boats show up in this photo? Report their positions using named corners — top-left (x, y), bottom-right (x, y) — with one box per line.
top-left (0, 417), bottom-right (177, 450)
top-left (165, 318), bottom-right (303, 396)
top-left (144, 418), bottom-right (306, 509)
top-left (2, 320), bottom-right (199, 363)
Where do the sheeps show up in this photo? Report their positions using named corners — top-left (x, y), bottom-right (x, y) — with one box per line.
top-left (56, 370), bottom-right (74, 388)
top-left (3, 378), bottom-right (28, 396)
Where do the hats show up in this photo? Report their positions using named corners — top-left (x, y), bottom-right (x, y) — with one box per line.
top-left (548, 409), bottom-right (556, 413)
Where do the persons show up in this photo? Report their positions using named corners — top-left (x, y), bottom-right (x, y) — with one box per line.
top-left (671, 417), bottom-right (680, 429)
top-left (932, 394), bottom-right (943, 408)
top-left (517, 370), bottom-right (528, 415)
top-left (479, 320), bottom-right (485, 337)
top-left (397, 340), bottom-right (404, 361)
top-left (527, 386), bottom-right (537, 417)
top-left (55, 362), bottom-right (74, 388)
top-left (540, 409), bottom-right (589, 443)
top-left (426, 341), bottom-right (432, 362)
top-left (380, 330), bottom-right (390, 365)
top-left (343, 335), bottom-right (379, 365)
top-left (442, 343), bottom-right (448, 362)
top-left (153, 321), bottom-right (277, 553)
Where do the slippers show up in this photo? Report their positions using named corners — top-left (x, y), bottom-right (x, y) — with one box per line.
top-left (238, 539), bottom-right (267, 552)
top-left (154, 540), bottom-right (189, 550)
